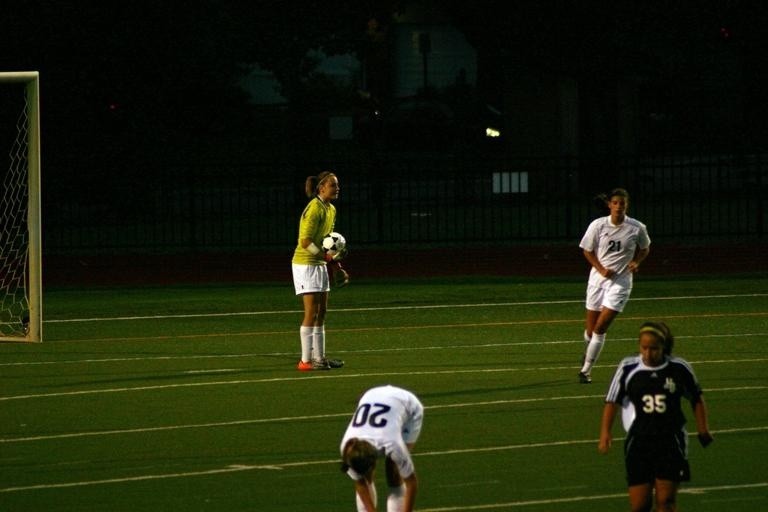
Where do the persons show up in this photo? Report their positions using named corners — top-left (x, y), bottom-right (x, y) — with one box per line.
top-left (290, 170), bottom-right (346, 371)
top-left (338, 383), bottom-right (424, 512)
top-left (599, 318), bottom-right (715, 512)
top-left (577, 189), bottom-right (653, 384)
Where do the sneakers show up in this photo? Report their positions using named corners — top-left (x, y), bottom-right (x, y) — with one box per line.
top-left (296, 356), bottom-right (345, 371)
top-left (579, 362), bottom-right (593, 383)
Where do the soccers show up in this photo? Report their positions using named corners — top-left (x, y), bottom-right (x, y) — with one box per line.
top-left (322, 233), bottom-right (346, 255)
